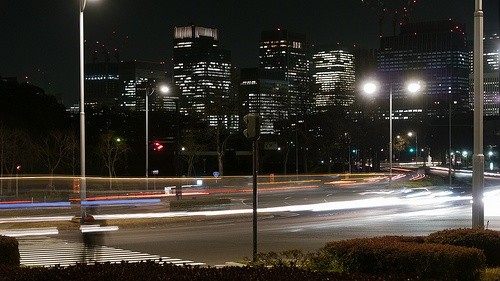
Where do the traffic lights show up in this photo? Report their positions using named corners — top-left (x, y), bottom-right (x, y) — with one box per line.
top-left (242, 113), bottom-right (248, 139)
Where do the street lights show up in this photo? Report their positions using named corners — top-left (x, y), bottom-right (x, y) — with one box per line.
top-left (146, 81), bottom-right (169, 177)
top-left (365, 80), bottom-right (392, 172)
top-left (408, 132), bottom-right (418, 162)
